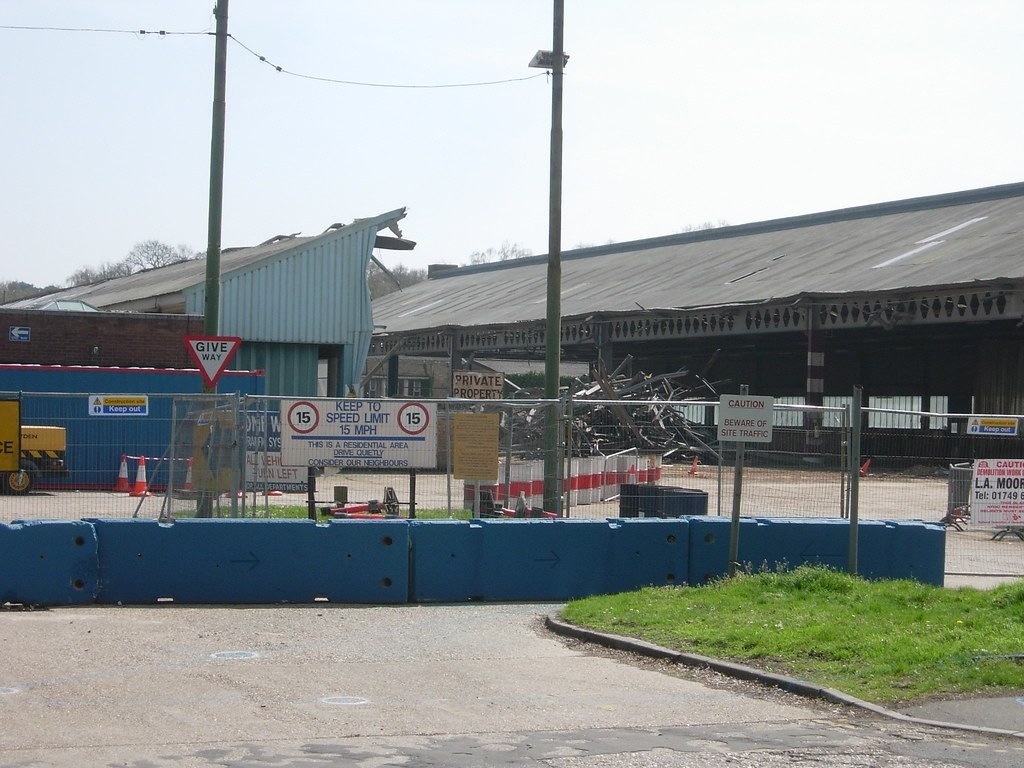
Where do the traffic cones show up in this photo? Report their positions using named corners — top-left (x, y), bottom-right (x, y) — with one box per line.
top-left (185, 456), bottom-right (193, 492)
top-left (114, 454), bottom-right (132, 492)
top-left (129, 456), bottom-right (152, 497)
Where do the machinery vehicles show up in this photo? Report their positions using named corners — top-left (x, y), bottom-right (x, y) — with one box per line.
top-left (0, 423), bottom-right (67, 493)
